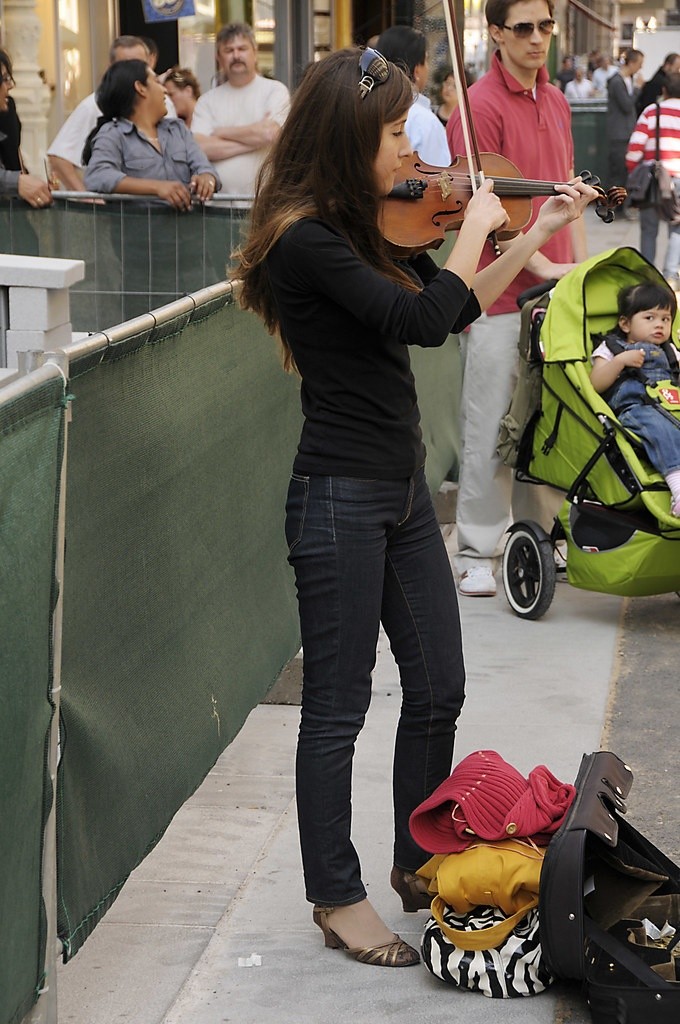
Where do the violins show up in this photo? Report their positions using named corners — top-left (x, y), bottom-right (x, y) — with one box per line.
top-left (378, 150), bottom-right (628, 261)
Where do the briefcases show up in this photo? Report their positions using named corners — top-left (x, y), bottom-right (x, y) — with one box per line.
top-left (539, 750), bottom-right (679, 1024)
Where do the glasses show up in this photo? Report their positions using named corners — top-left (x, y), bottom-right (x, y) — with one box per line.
top-left (356, 47), bottom-right (390, 100)
top-left (499, 18), bottom-right (556, 38)
top-left (0, 73), bottom-right (11, 84)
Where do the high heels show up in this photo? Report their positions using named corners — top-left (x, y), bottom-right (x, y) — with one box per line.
top-left (312, 900), bottom-right (420, 968)
top-left (390, 864), bottom-right (439, 914)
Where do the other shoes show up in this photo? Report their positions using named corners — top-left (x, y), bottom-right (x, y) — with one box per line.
top-left (665, 276), bottom-right (680, 292)
top-left (669, 489), bottom-right (680, 517)
top-left (458, 566), bottom-right (496, 596)
top-left (552, 549), bottom-right (570, 584)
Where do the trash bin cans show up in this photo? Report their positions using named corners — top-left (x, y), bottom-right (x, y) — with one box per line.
top-left (569, 100), bottom-right (610, 193)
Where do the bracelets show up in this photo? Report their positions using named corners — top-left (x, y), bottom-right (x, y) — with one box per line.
top-left (207, 179), bottom-right (216, 186)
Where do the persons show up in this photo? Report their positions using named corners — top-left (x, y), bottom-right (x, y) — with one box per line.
top-left (49, 36), bottom-right (199, 192)
top-left (364, 28), bottom-right (476, 165)
top-left (548, 43), bottom-right (679, 209)
top-left (229, 48), bottom-right (601, 969)
top-left (626, 73), bottom-right (679, 289)
top-left (85, 59), bottom-right (224, 212)
top-left (191, 23), bottom-right (292, 196)
top-left (590, 277), bottom-right (679, 530)
top-left (0, 41), bottom-right (56, 208)
top-left (446, 0), bottom-right (589, 597)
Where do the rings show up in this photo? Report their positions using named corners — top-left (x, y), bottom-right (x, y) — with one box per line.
top-left (36, 197), bottom-right (41, 201)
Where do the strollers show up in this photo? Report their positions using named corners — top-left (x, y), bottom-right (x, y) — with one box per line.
top-left (502, 245), bottom-right (680, 615)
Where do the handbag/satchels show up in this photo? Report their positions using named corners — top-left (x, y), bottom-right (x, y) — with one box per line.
top-left (417, 829), bottom-right (553, 999)
top-left (624, 163), bottom-right (662, 208)
